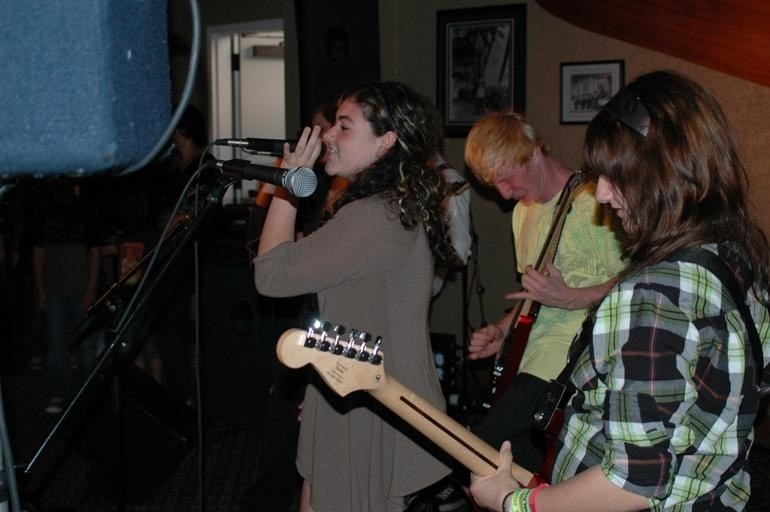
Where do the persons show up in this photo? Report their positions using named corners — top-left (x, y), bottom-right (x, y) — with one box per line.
top-left (463, 112), bottom-right (633, 474)
top-left (468, 68), bottom-right (769, 511)
top-left (28, 80), bottom-right (472, 512)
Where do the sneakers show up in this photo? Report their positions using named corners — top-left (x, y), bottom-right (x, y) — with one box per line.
top-left (434, 487), bottom-right (469, 512)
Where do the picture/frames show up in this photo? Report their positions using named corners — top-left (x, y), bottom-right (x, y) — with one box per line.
top-left (435, 2), bottom-right (529, 139)
top-left (558, 59), bottom-right (626, 125)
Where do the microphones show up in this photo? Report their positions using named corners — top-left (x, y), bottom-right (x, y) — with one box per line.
top-left (215, 158), bottom-right (318, 198)
top-left (214, 137), bottom-right (327, 162)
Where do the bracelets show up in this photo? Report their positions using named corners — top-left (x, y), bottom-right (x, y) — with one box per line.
top-left (510, 482), bottom-right (551, 512)
top-left (502, 490), bottom-right (513, 511)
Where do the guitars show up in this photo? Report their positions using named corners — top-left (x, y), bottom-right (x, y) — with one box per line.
top-left (478, 170), bottom-right (585, 423)
